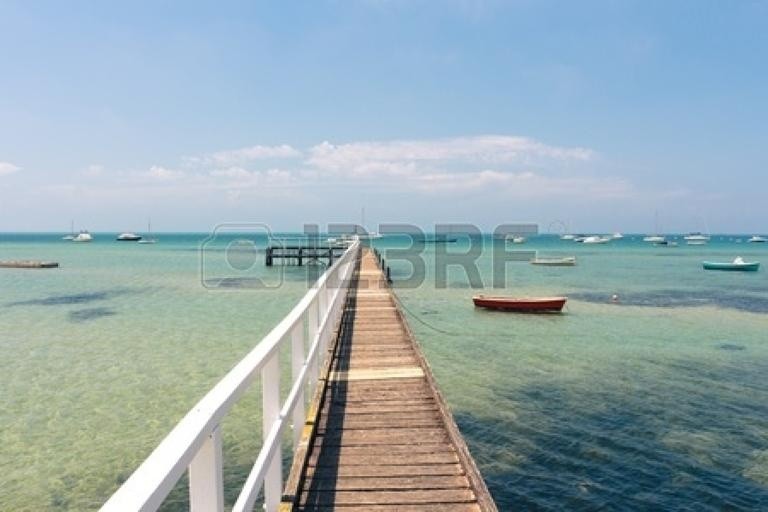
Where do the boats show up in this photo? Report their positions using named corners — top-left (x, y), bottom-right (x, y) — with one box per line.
top-left (614, 234), bottom-right (624, 239)
top-left (531, 256), bottom-right (575, 265)
top-left (472, 295), bottom-right (568, 311)
top-left (326, 232), bottom-right (383, 245)
top-left (643, 237), bottom-right (665, 242)
top-left (701, 260), bottom-right (760, 272)
top-left (511, 235), bottom-right (524, 243)
top-left (562, 235), bottom-right (573, 240)
top-left (685, 232), bottom-right (709, 244)
top-left (116, 232), bottom-right (142, 240)
top-left (62, 236), bottom-right (73, 240)
top-left (73, 231), bottom-right (93, 241)
top-left (575, 236), bottom-right (608, 244)
top-left (749, 236), bottom-right (765, 241)
